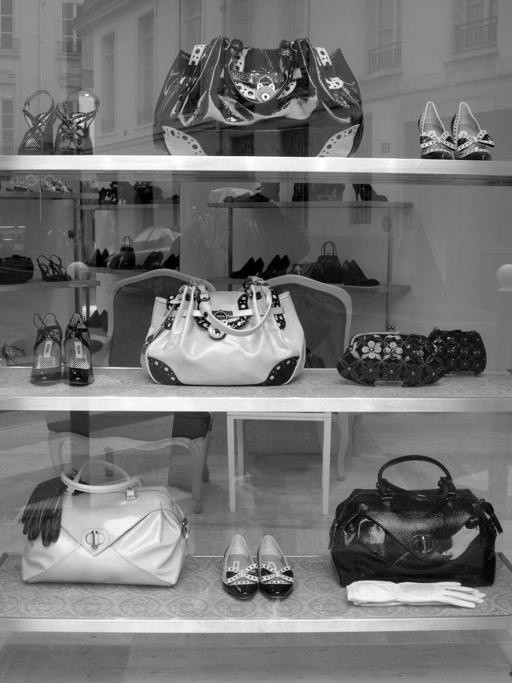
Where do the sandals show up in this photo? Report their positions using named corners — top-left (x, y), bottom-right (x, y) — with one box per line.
top-left (38, 253), bottom-right (71, 282)
top-left (18, 89), bottom-right (101, 153)
top-left (5, 175), bottom-right (75, 192)
top-left (99, 181), bottom-right (166, 205)
top-left (417, 101), bottom-right (494, 159)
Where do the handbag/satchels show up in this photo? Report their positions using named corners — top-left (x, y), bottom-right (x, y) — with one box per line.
top-left (337, 330), bottom-right (486, 386)
top-left (0, 255), bottom-right (34, 283)
top-left (21, 462), bottom-right (189, 587)
top-left (108, 246), bottom-right (136, 269)
top-left (153, 36), bottom-right (362, 157)
top-left (329, 456), bottom-right (502, 590)
top-left (141, 276), bottom-right (306, 388)
top-left (291, 254), bottom-right (342, 283)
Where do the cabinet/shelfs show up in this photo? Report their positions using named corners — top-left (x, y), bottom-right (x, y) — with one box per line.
top-left (81, 203), bottom-right (178, 336)
top-left (1, 154), bottom-right (512, 633)
top-left (1, 190), bottom-right (110, 366)
top-left (207, 202), bottom-right (411, 331)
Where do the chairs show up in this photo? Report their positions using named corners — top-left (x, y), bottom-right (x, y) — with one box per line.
top-left (236, 275), bottom-right (362, 480)
top-left (48, 269), bottom-right (215, 513)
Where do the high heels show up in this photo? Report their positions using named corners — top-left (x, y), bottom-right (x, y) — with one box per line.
top-left (2, 314), bottom-right (94, 386)
top-left (352, 183), bottom-right (388, 201)
top-left (231, 255), bottom-right (289, 281)
top-left (137, 251), bottom-right (179, 271)
top-left (86, 249), bottom-right (109, 266)
top-left (344, 260), bottom-right (379, 287)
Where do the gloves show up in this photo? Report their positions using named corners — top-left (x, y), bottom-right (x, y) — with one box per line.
top-left (347, 580), bottom-right (485, 607)
top-left (22, 476), bottom-right (89, 546)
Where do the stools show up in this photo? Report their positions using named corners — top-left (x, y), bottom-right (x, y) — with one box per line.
top-left (226, 412), bottom-right (332, 515)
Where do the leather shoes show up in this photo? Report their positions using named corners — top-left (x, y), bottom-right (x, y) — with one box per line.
top-left (85, 310), bottom-right (108, 331)
top-left (221, 534), bottom-right (292, 599)
top-left (224, 191), bottom-right (269, 202)
